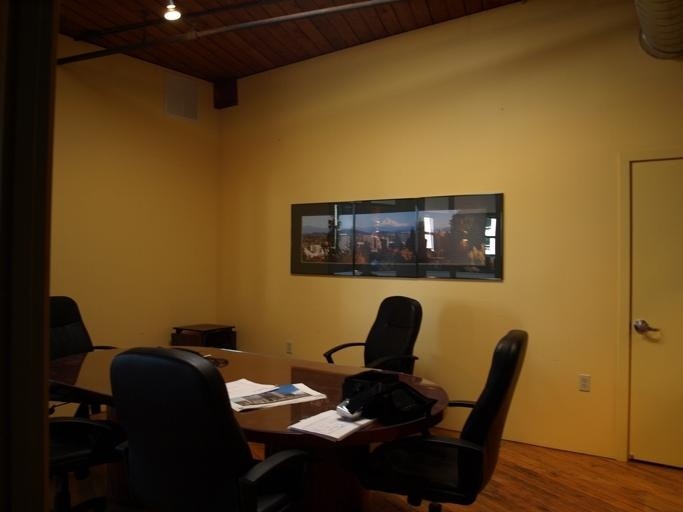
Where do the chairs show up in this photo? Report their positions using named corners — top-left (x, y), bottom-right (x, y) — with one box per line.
top-left (351, 328), bottom-right (529, 511)
top-left (321, 295), bottom-right (424, 374)
top-left (104, 346), bottom-right (309, 511)
top-left (46, 295), bottom-right (110, 434)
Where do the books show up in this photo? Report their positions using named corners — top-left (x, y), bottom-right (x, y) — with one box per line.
top-left (223, 376), bottom-right (327, 413)
top-left (286, 408), bottom-right (377, 443)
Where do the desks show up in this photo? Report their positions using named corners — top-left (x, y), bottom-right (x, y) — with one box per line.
top-left (51, 343), bottom-right (447, 512)
